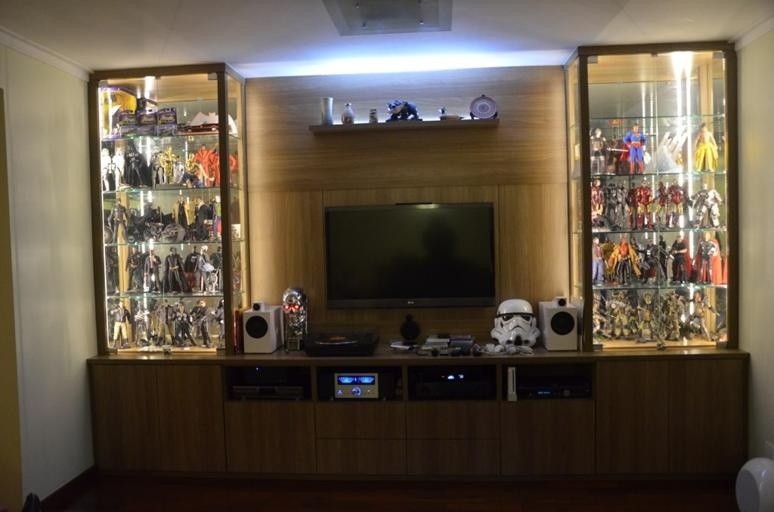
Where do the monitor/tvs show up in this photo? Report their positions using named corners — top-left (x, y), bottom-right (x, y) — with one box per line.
top-left (324, 201), bottom-right (500, 308)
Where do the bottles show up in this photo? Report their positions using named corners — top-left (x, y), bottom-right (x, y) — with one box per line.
top-left (341, 102), bottom-right (356, 124)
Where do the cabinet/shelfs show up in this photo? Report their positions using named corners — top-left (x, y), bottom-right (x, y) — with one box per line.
top-left (563, 39), bottom-right (739, 352)
top-left (597, 350), bottom-right (750, 485)
top-left (88, 62), bottom-right (251, 355)
top-left (86, 354), bottom-right (226, 485)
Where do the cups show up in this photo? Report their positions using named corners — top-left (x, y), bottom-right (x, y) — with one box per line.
top-left (320, 96), bottom-right (333, 126)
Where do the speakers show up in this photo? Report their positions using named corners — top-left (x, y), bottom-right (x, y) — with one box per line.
top-left (538, 296), bottom-right (578, 352)
top-left (241, 301), bottom-right (281, 354)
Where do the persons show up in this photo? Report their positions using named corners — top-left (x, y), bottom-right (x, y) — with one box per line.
top-left (592, 288), bottom-right (721, 341)
top-left (592, 234), bottom-right (722, 283)
top-left (123, 247), bottom-right (241, 293)
top-left (590, 180), bottom-right (724, 227)
top-left (110, 299), bottom-right (225, 348)
top-left (104, 139), bottom-right (236, 186)
top-left (590, 119), bottom-right (723, 173)
top-left (105, 199), bottom-right (222, 240)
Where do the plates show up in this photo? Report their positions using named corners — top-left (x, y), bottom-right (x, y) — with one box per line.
top-left (470, 96), bottom-right (498, 119)
